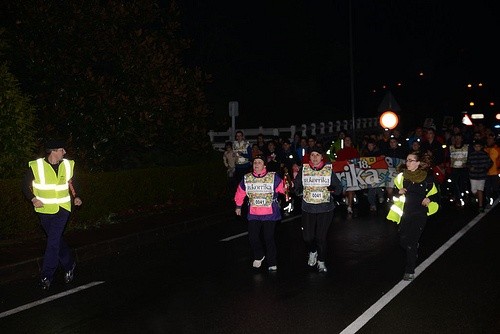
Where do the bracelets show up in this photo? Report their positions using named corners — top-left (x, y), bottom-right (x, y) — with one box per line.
top-left (74, 195), bottom-right (79, 199)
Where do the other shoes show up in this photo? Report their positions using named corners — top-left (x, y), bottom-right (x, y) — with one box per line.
top-left (347, 206), bottom-right (353, 214)
top-left (268, 265), bottom-right (277, 271)
top-left (478, 207), bottom-right (485, 214)
top-left (307, 250), bottom-right (318, 267)
top-left (63, 261), bottom-right (76, 284)
top-left (252, 256), bottom-right (265, 268)
top-left (401, 272), bottom-right (412, 280)
top-left (317, 260), bottom-right (327, 273)
top-left (38, 275), bottom-right (53, 290)
top-left (370, 206), bottom-right (376, 211)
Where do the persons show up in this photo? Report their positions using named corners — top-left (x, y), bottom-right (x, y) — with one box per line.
top-left (389, 150), bottom-right (440, 281)
top-left (224, 120), bottom-right (500, 214)
top-left (294, 146), bottom-right (343, 272)
top-left (233, 153), bottom-right (289, 271)
top-left (24, 136), bottom-right (83, 289)
top-left (466, 139), bottom-right (493, 213)
